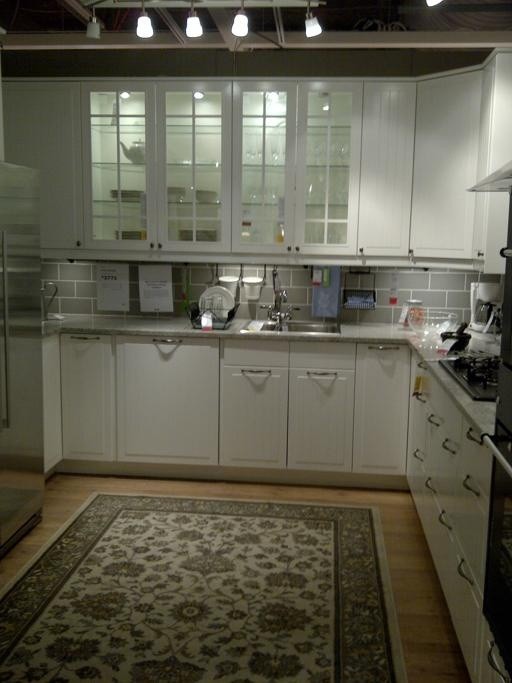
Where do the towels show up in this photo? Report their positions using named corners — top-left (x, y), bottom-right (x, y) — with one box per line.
top-left (343, 291), bottom-right (375, 310)
top-left (310, 264), bottom-right (342, 319)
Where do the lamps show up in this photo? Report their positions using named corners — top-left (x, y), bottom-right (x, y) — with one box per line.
top-left (83, 0), bottom-right (324, 40)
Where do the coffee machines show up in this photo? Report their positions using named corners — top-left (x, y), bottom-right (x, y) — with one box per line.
top-left (467, 280), bottom-right (502, 333)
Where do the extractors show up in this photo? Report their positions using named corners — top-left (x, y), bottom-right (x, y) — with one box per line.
top-left (466, 158), bottom-right (512, 193)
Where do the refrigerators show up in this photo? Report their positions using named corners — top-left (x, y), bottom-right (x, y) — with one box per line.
top-left (1, 160), bottom-right (47, 564)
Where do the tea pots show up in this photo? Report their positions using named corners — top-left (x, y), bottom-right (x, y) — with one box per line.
top-left (120, 138), bottom-right (147, 164)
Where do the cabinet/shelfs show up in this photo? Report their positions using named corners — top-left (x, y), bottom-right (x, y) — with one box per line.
top-left (472, 45), bottom-right (511, 274)
top-left (221, 337), bottom-right (288, 470)
top-left (406, 346), bottom-right (512, 680)
top-left (234, 79), bottom-right (350, 262)
top-left (355, 75), bottom-right (419, 269)
top-left (86, 79), bottom-right (231, 253)
top-left (116, 331), bottom-right (218, 473)
top-left (42, 332), bottom-right (114, 474)
top-left (352, 341), bottom-right (406, 478)
top-left (289, 340), bottom-right (356, 474)
top-left (6, 78), bottom-right (85, 250)
top-left (407, 60), bottom-right (477, 273)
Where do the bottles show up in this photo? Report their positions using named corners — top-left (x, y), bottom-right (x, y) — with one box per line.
top-left (398, 300), bottom-right (424, 326)
top-left (276, 222), bottom-right (284, 243)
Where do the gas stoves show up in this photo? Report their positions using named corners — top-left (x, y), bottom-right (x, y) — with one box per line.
top-left (438, 357), bottom-right (500, 401)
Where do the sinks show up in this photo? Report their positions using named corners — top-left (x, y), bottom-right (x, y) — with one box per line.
top-left (237, 319), bottom-right (285, 336)
top-left (284, 319), bottom-right (343, 337)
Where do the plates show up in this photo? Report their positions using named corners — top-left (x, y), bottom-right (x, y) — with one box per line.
top-left (116, 229), bottom-right (147, 239)
top-left (198, 286), bottom-right (233, 317)
top-left (111, 188), bottom-right (142, 200)
top-left (179, 227), bottom-right (217, 241)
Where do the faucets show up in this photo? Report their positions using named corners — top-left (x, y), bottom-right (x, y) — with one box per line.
top-left (272, 289), bottom-right (288, 322)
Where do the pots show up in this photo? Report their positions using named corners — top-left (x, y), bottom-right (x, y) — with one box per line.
top-left (440, 318), bottom-right (470, 350)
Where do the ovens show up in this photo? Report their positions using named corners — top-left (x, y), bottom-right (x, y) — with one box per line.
top-left (482, 187), bottom-right (512, 676)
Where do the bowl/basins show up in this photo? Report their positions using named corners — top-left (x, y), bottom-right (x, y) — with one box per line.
top-left (197, 190), bottom-right (216, 202)
top-left (168, 186), bottom-right (186, 201)
top-left (406, 309), bottom-right (459, 348)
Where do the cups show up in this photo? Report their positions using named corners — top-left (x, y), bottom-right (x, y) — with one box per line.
top-left (219, 275), bottom-right (240, 297)
top-left (242, 276), bottom-right (263, 300)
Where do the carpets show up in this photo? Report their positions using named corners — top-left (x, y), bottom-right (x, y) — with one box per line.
top-left (0, 489), bottom-right (410, 678)
